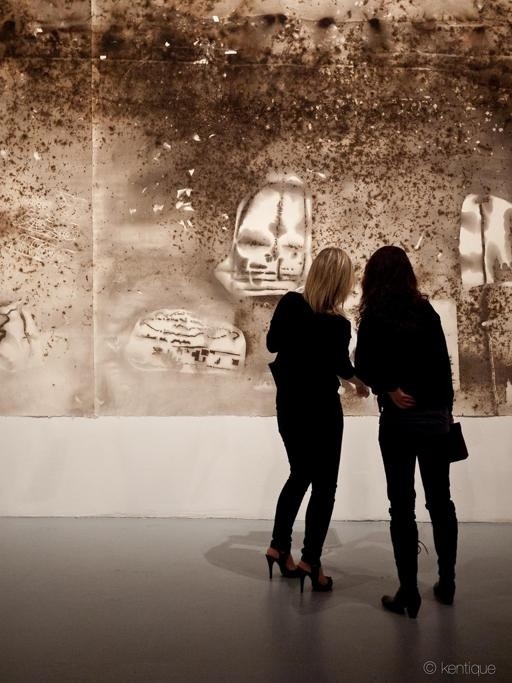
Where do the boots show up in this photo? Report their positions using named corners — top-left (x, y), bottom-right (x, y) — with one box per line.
top-left (382, 528), bottom-right (428, 618)
top-left (434, 519), bottom-right (458, 603)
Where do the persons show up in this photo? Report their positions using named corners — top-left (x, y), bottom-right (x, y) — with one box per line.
top-left (353, 245), bottom-right (468, 620)
top-left (264, 247), bottom-right (370, 594)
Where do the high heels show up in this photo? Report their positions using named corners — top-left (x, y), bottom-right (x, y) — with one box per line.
top-left (297, 557), bottom-right (332, 593)
top-left (265, 543), bottom-right (298, 579)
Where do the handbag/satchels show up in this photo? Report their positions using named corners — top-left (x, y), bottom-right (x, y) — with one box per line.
top-left (449, 422), bottom-right (469, 464)
